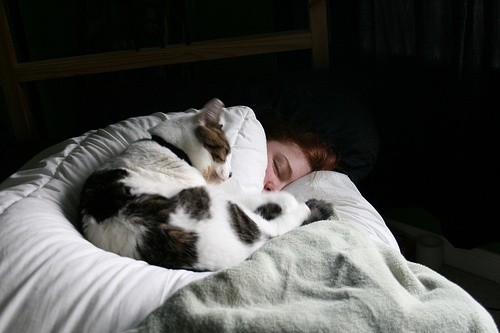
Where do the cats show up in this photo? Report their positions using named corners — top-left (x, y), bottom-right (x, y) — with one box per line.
top-left (80, 99), bottom-right (334, 273)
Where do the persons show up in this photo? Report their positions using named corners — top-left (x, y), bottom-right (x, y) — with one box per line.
top-left (262, 127), bottom-right (339, 194)
